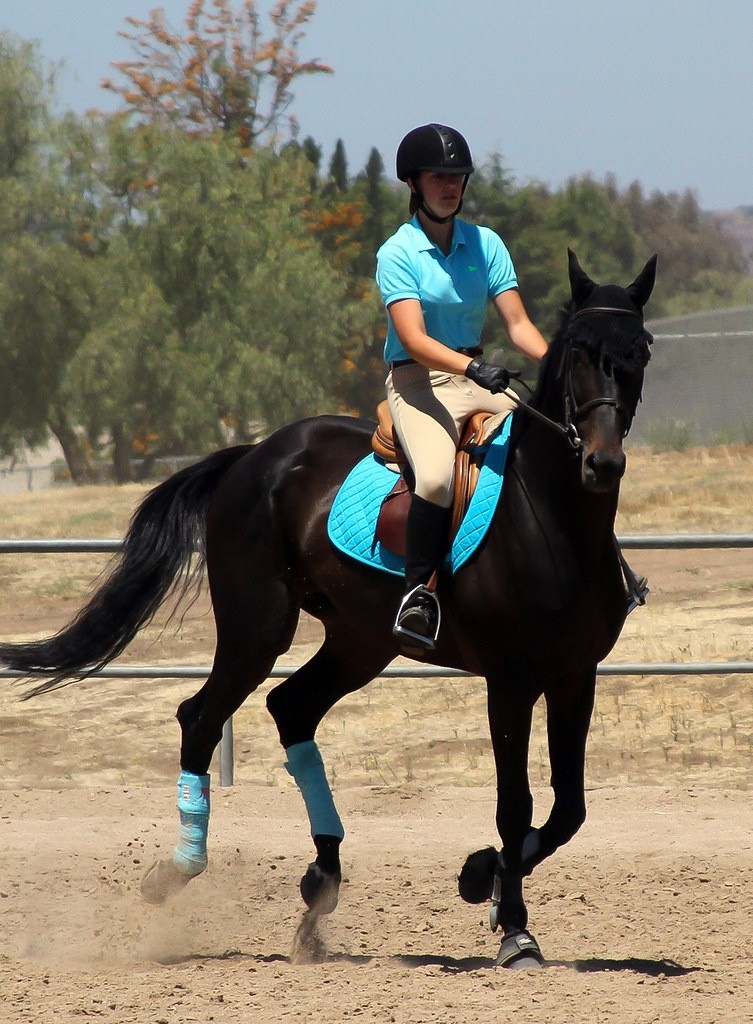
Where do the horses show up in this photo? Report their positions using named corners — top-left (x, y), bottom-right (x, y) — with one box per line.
top-left (0, 248), bottom-right (660, 973)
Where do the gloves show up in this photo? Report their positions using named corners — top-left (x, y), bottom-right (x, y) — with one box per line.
top-left (464, 357), bottom-right (521, 394)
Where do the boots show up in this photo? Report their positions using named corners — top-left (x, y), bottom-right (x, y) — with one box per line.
top-left (398, 491), bottom-right (454, 650)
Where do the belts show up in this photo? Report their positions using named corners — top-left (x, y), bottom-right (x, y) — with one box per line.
top-left (388, 346), bottom-right (483, 371)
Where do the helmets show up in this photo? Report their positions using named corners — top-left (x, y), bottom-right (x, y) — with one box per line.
top-left (397, 123), bottom-right (474, 180)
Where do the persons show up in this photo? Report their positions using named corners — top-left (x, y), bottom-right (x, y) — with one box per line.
top-left (375, 123), bottom-right (548, 647)
